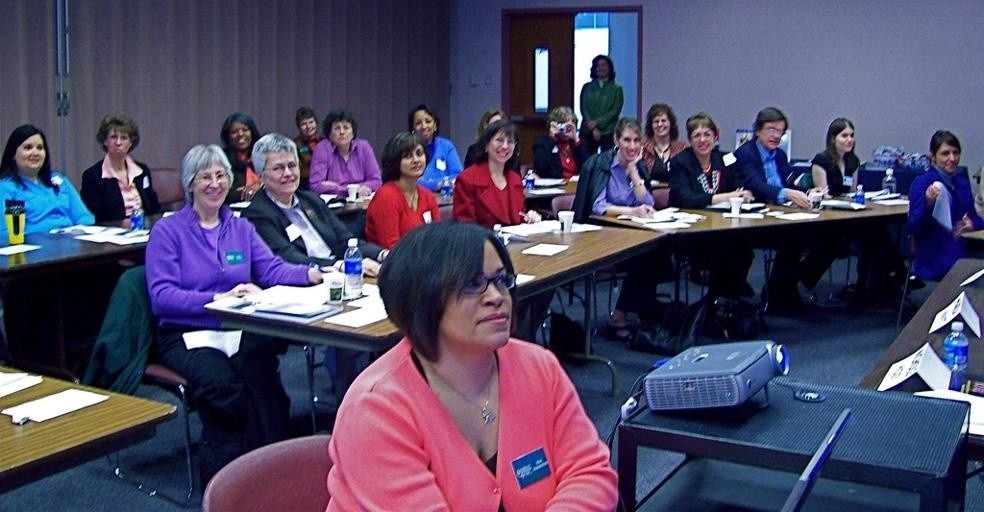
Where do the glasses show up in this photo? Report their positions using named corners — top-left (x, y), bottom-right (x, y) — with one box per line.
top-left (190, 175), bottom-right (230, 183)
top-left (761, 125), bottom-right (786, 136)
top-left (261, 162), bottom-right (297, 172)
top-left (447, 274), bottom-right (515, 295)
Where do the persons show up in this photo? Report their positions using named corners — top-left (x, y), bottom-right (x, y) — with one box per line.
top-left (578, 56), bottom-right (624, 153)
top-left (2, 103), bottom-right (984, 402)
top-left (329, 220), bottom-right (619, 512)
top-left (145, 143), bottom-right (336, 468)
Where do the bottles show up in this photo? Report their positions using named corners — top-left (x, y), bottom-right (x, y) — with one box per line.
top-left (439, 176), bottom-right (450, 200)
top-left (523, 169), bottom-right (533, 192)
top-left (854, 185), bottom-right (865, 206)
top-left (129, 203), bottom-right (144, 232)
top-left (343, 237), bottom-right (363, 300)
top-left (491, 222), bottom-right (503, 241)
top-left (942, 321), bottom-right (971, 390)
top-left (3, 198), bottom-right (24, 245)
top-left (881, 168), bottom-right (897, 194)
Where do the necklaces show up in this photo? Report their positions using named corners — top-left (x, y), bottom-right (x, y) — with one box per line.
top-left (430, 364), bottom-right (497, 424)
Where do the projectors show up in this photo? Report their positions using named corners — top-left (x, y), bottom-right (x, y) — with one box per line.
top-left (646, 340), bottom-right (790, 414)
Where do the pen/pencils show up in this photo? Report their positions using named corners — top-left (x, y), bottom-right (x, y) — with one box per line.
top-left (519, 211), bottom-right (527, 217)
top-left (960, 380), bottom-right (971, 394)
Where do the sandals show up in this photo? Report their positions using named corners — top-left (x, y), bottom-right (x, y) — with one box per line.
top-left (608, 323), bottom-right (638, 344)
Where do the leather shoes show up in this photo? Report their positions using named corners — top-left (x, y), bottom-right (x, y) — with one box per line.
top-left (709, 290), bottom-right (816, 316)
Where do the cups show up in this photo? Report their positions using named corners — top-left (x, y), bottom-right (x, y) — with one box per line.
top-left (729, 197), bottom-right (743, 217)
top-left (557, 210), bottom-right (574, 234)
top-left (810, 193), bottom-right (822, 214)
top-left (322, 273), bottom-right (345, 306)
